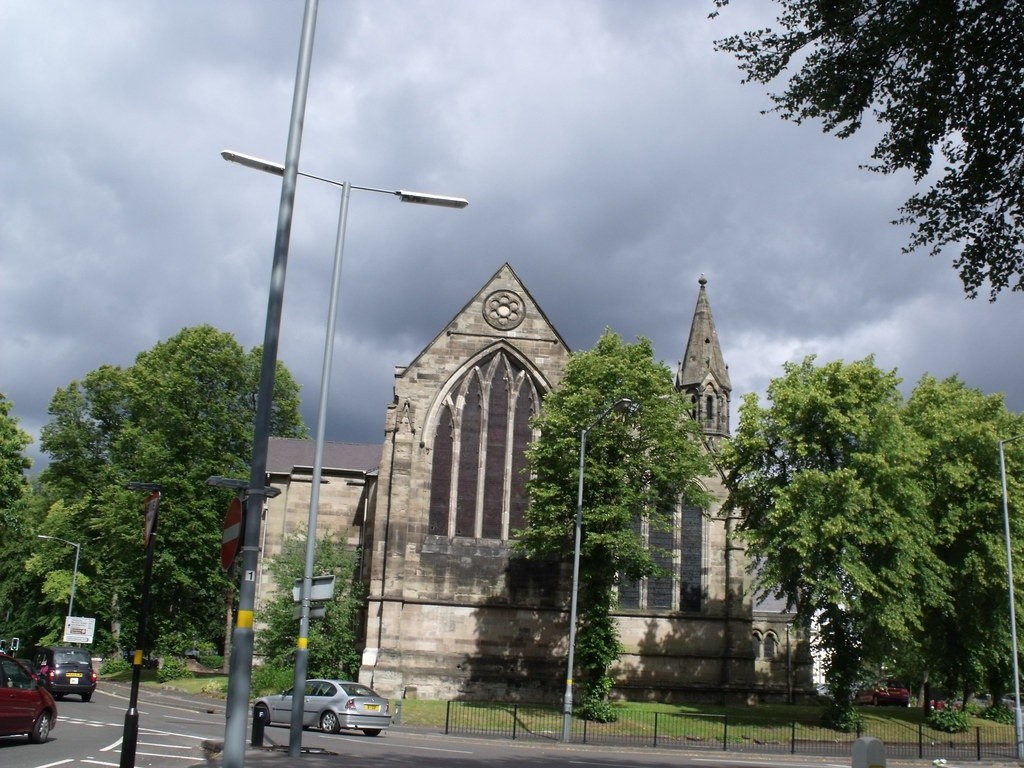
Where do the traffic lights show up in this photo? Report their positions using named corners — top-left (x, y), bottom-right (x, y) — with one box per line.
top-left (1, 640), bottom-right (6, 651)
top-left (11, 638), bottom-right (19, 651)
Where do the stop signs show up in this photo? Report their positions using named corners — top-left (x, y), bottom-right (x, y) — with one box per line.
top-left (222, 497), bottom-right (242, 570)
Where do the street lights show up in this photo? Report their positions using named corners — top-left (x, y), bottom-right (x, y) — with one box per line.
top-left (998, 435), bottom-right (1023, 766)
top-left (562, 398), bottom-right (633, 743)
top-left (222, 150), bottom-right (469, 758)
top-left (38, 535), bottom-right (80, 647)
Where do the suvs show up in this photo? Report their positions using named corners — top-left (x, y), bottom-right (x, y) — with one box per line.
top-left (33, 647), bottom-right (97, 702)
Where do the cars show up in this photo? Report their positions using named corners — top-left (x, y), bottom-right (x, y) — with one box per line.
top-left (252, 679), bottom-right (392, 737)
top-left (930, 693), bottom-right (1024, 726)
top-left (0, 650), bottom-right (57, 743)
top-left (817, 675), bottom-right (909, 708)
